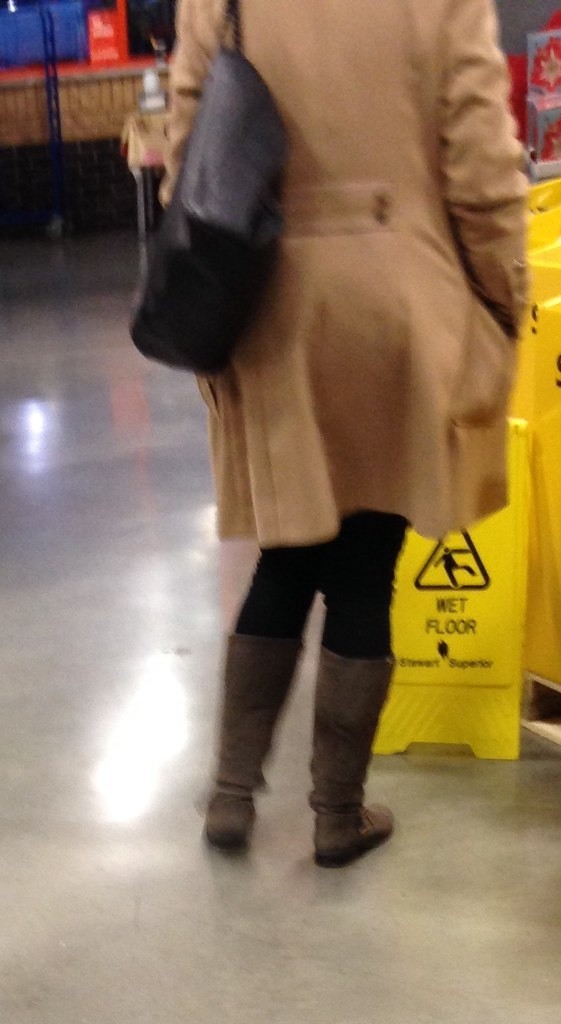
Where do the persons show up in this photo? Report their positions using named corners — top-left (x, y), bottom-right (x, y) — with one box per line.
top-left (125, 1), bottom-right (529, 869)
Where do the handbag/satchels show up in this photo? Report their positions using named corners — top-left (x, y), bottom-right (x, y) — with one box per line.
top-left (128, 44), bottom-right (291, 378)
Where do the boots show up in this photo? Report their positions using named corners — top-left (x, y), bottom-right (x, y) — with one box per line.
top-left (308, 641), bottom-right (396, 871)
top-left (202, 631), bottom-right (305, 849)
